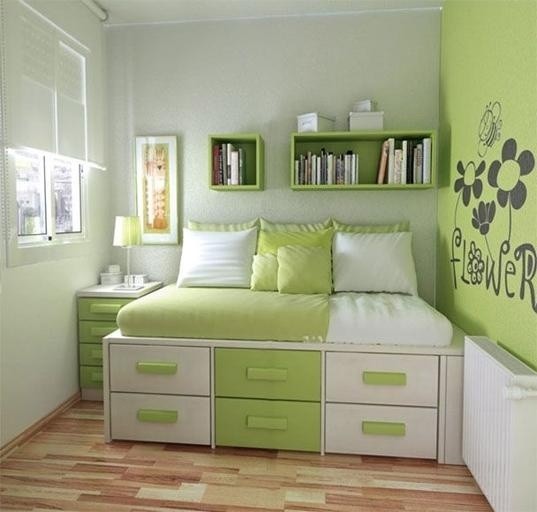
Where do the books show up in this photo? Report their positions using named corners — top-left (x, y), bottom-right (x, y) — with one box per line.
top-left (377, 138), bottom-right (431, 184)
top-left (293, 146), bottom-right (359, 186)
top-left (211, 142), bottom-right (246, 186)
top-left (113, 284), bottom-right (143, 291)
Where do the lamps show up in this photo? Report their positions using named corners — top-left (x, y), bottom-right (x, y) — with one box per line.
top-left (112, 214), bottom-right (142, 287)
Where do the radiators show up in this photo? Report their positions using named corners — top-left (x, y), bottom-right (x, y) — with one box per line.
top-left (456, 330), bottom-right (537, 511)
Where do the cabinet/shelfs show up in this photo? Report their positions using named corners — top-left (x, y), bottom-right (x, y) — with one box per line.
top-left (289, 130), bottom-right (437, 191)
top-left (207, 133), bottom-right (265, 191)
top-left (75, 281), bottom-right (164, 405)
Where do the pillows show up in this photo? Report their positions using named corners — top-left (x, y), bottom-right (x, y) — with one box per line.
top-left (174, 216), bottom-right (423, 300)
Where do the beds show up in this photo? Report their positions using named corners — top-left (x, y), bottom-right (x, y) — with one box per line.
top-left (101, 221), bottom-right (466, 467)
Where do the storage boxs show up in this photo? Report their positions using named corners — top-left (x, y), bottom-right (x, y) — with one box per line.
top-left (353, 99), bottom-right (378, 112)
top-left (346, 112), bottom-right (385, 131)
top-left (296, 112), bottom-right (336, 132)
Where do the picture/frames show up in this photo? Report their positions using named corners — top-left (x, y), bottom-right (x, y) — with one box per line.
top-left (129, 132), bottom-right (182, 247)
